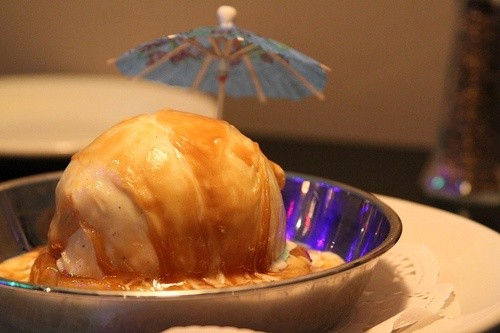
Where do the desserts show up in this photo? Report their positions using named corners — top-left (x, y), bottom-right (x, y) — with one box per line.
top-left (0, 108), bottom-right (349, 292)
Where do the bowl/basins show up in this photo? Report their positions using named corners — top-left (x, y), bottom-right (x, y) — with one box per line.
top-left (0, 174), bottom-right (402, 333)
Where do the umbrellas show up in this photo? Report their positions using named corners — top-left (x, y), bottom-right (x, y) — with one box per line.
top-left (106, 5), bottom-right (331, 120)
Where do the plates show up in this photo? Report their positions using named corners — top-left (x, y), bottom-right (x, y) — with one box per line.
top-left (328, 192), bottom-right (500, 333)
top-left (0, 75), bottom-right (218, 158)
